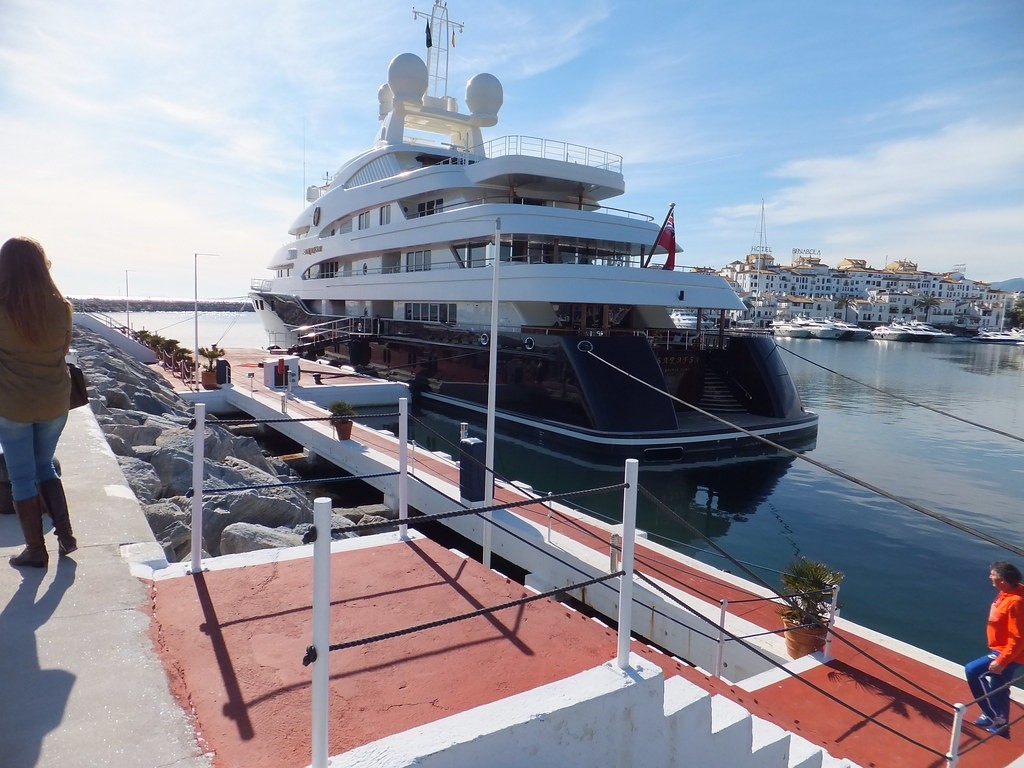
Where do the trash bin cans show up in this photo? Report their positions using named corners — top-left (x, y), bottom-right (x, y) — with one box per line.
top-left (459, 437), bottom-right (494, 501)
top-left (215, 358), bottom-right (231, 384)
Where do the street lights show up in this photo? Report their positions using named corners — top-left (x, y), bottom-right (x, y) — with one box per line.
top-left (126, 270), bottom-right (136, 337)
top-left (194, 253), bottom-right (219, 391)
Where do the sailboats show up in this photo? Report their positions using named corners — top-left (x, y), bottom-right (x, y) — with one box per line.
top-left (733, 199), bottom-right (773, 335)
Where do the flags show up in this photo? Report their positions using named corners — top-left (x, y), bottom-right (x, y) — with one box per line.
top-left (658, 211), bottom-right (676, 270)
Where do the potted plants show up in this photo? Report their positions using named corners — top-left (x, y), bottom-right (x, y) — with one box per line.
top-left (773, 556), bottom-right (846, 661)
top-left (132, 330), bottom-right (226, 390)
top-left (328, 400), bottom-right (358, 441)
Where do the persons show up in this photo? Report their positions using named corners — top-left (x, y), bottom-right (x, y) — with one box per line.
top-left (963, 561), bottom-right (1024, 737)
top-left (0, 236), bottom-right (78, 568)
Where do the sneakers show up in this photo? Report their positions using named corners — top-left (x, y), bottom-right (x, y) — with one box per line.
top-left (974, 715), bottom-right (1006, 726)
top-left (985, 726), bottom-right (1010, 740)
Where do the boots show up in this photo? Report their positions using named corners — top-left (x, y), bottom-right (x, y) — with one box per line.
top-left (38, 478), bottom-right (78, 557)
top-left (9, 494), bottom-right (50, 569)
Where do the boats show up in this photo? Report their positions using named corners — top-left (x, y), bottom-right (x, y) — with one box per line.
top-left (670, 309), bottom-right (714, 329)
top-left (825, 316), bottom-right (1024, 344)
top-left (248, 0), bottom-right (819, 474)
top-left (790, 313), bottom-right (846, 339)
top-left (769, 319), bottom-right (809, 337)
